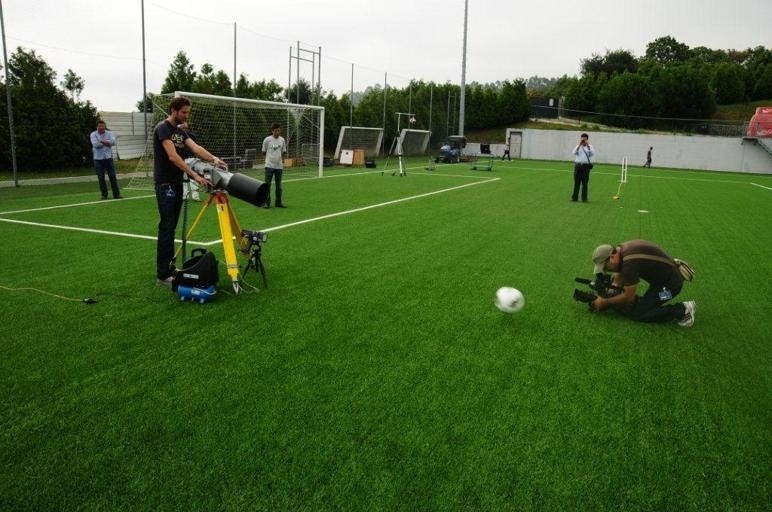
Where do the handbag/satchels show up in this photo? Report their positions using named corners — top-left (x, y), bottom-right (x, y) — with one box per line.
top-left (673, 258), bottom-right (695, 281)
top-left (172, 252), bottom-right (219, 292)
top-left (587, 163), bottom-right (593, 169)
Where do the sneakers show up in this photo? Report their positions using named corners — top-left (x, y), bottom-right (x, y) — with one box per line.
top-left (678, 300), bottom-right (696, 327)
top-left (156, 268), bottom-right (181, 288)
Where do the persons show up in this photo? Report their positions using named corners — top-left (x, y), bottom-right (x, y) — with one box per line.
top-left (592, 238), bottom-right (696, 328)
top-left (180, 121), bottom-right (203, 201)
top-left (643, 146), bottom-right (653, 168)
top-left (502, 137), bottom-right (512, 161)
top-left (261, 123), bottom-right (288, 208)
top-left (153, 96), bottom-right (228, 286)
top-left (90, 121), bottom-right (124, 200)
top-left (570, 133), bottom-right (594, 202)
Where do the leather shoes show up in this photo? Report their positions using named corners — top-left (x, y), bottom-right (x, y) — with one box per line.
top-left (101, 195), bottom-right (108, 200)
top-left (275, 204), bottom-right (286, 208)
top-left (263, 202), bottom-right (269, 208)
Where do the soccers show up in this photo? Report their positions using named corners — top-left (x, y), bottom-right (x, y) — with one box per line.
top-left (494, 287), bottom-right (525, 315)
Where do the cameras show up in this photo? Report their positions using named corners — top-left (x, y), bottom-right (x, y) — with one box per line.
top-left (581, 140), bottom-right (585, 144)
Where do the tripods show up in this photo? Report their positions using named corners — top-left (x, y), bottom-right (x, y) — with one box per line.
top-left (171, 190), bottom-right (249, 296)
top-left (382, 132), bottom-right (407, 179)
top-left (239, 251), bottom-right (269, 288)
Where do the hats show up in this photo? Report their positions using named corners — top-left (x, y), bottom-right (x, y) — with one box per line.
top-left (592, 244), bottom-right (614, 274)
